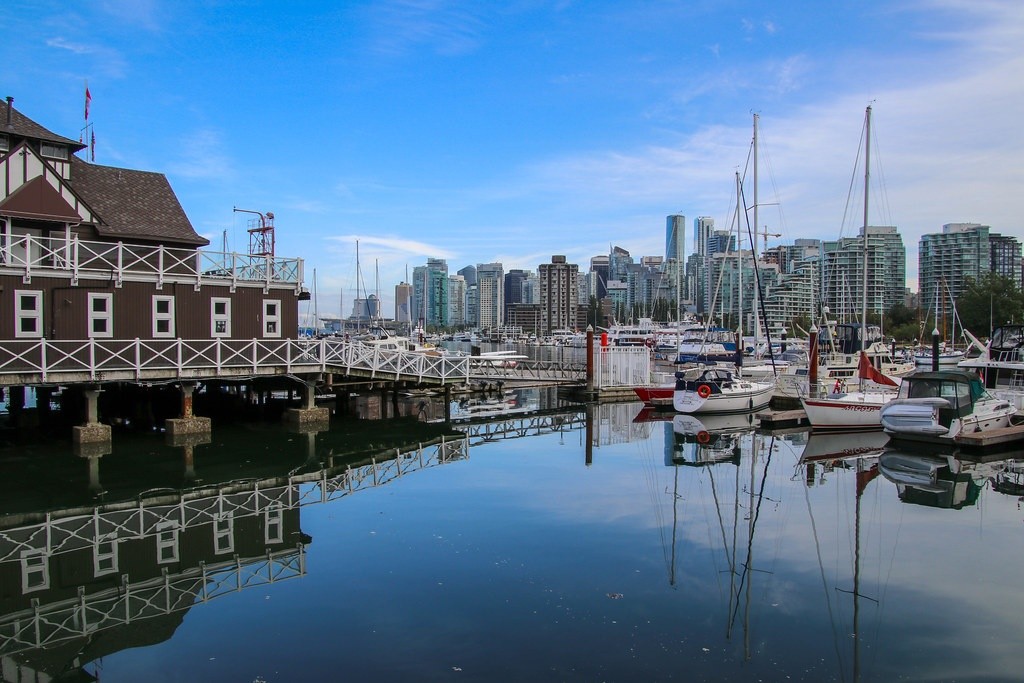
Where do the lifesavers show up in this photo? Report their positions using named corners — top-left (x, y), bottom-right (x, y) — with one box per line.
top-left (698, 385), bottom-right (711, 398)
top-left (697, 431), bottom-right (710, 444)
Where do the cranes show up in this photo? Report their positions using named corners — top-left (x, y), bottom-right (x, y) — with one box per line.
top-left (728, 225), bottom-right (782, 252)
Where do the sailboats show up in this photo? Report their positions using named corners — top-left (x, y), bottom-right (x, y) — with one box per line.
top-left (876, 438), bottom-right (1024, 511)
top-left (794, 105), bottom-right (909, 432)
top-left (298, 238), bottom-right (1024, 418)
top-left (631, 405), bottom-right (694, 587)
top-left (672, 413), bottom-right (808, 664)
top-left (789, 427), bottom-right (892, 683)
top-left (673, 113), bottom-right (793, 414)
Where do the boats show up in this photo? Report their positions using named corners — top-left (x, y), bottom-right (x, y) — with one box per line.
top-left (877, 251), bottom-right (1017, 446)
